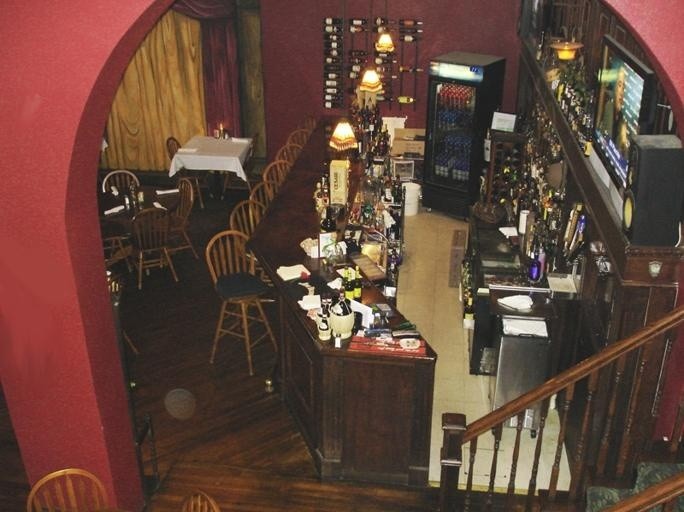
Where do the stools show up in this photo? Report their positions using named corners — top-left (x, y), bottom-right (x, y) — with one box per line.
top-left (203, 119), bottom-right (317, 377)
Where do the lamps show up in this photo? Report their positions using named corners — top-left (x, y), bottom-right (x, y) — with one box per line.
top-left (375, 34), bottom-right (395, 65)
top-left (328, 116), bottom-right (358, 160)
top-left (360, 69), bottom-right (382, 111)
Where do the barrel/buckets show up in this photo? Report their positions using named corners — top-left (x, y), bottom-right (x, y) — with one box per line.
top-left (402, 182), bottom-right (423, 217)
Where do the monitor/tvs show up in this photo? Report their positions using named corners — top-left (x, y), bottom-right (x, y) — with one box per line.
top-left (590, 32), bottom-right (657, 201)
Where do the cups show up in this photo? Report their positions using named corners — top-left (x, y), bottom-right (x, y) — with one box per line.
top-left (649, 262), bottom-right (663, 278)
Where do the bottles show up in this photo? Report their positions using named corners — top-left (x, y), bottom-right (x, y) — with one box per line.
top-left (128, 180), bottom-right (138, 207)
top-left (530, 246), bottom-right (546, 283)
top-left (461, 258), bottom-right (474, 320)
top-left (314, 16), bottom-right (425, 234)
top-left (335, 333), bottom-right (342, 349)
top-left (558, 80), bottom-right (594, 158)
top-left (343, 265), bottom-right (362, 301)
top-left (435, 84), bottom-right (472, 179)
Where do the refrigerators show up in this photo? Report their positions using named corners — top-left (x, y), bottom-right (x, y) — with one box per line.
top-left (423, 49), bottom-right (507, 214)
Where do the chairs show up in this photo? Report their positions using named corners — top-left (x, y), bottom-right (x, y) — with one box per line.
top-left (102, 131), bottom-right (261, 358)
top-left (176, 490), bottom-right (221, 512)
top-left (25, 468), bottom-right (110, 512)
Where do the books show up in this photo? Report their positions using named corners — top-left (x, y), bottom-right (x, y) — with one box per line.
top-left (345, 336), bottom-right (427, 358)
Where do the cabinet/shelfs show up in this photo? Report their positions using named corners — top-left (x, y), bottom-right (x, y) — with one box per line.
top-left (461, 1), bottom-right (684, 481)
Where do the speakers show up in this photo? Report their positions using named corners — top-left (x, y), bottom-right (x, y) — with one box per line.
top-left (622, 134), bottom-right (684, 247)
